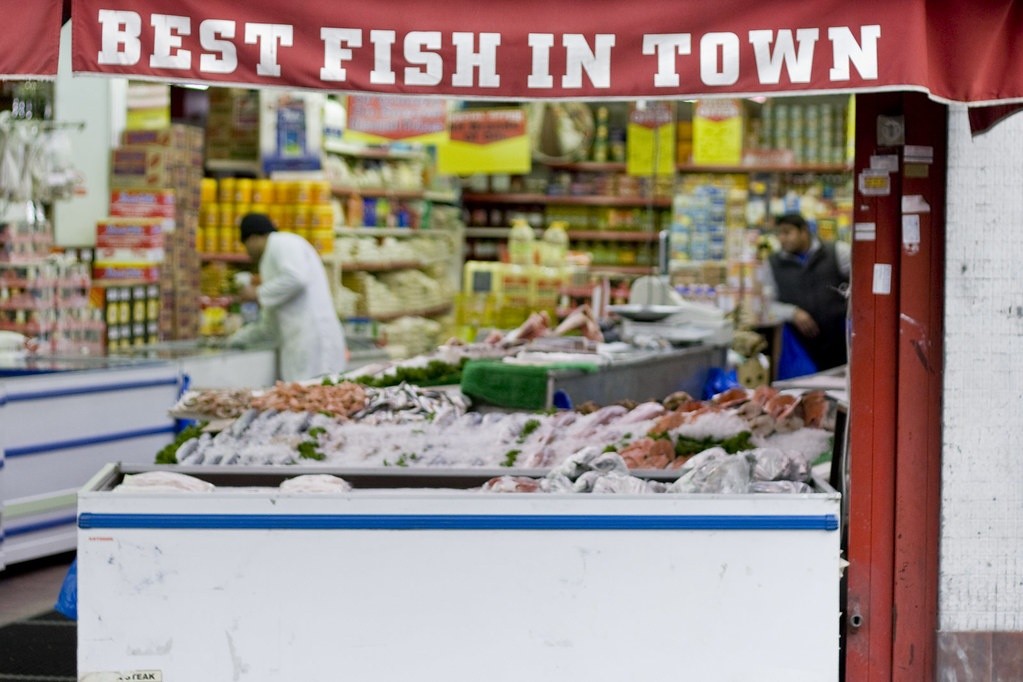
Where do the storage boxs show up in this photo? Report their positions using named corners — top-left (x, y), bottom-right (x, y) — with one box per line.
top-left (84, 126), bottom-right (197, 354)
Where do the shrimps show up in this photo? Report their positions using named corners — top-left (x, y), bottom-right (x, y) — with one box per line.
top-left (180, 375), bottom-right (365, 417)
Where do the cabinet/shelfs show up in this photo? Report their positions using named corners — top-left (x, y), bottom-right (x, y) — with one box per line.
top-left (199, 155), bottom-right (853, 351)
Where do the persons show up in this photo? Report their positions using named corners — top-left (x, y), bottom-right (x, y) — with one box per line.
top-left (751, 212), bottom-right (851, 374)
top-left (236, 211), bottom-right (345, 383)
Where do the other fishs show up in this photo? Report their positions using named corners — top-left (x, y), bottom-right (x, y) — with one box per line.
top-left (173, 406), bottom-right (330, 466)
top-left (348, 379), bottom-right (471, 424)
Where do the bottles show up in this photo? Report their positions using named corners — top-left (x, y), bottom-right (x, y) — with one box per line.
top-left (505, 217), bottom-right (593, 316)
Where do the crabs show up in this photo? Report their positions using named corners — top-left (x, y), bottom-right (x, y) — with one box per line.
top-left (482, 384), bottom-right (826, 491)
